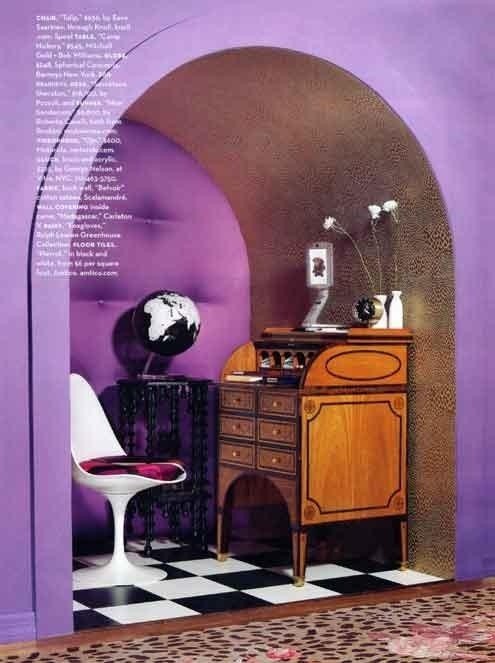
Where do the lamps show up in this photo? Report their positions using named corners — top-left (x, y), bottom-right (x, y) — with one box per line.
top-left (299, 240), bottom-right (350, 329)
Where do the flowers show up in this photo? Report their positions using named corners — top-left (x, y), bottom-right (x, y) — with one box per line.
top-left (323, 200), bottom-right (401, 295)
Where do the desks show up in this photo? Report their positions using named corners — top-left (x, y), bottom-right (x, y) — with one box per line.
top-left (116, 376), bottom-right (214, 559)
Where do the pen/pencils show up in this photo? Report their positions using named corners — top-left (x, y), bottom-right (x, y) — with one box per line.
top-left (297, 327), bottom-right (337, 332)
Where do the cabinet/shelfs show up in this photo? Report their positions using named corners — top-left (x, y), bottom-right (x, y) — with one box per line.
top-left (213, 325), bottom-right (414, 588)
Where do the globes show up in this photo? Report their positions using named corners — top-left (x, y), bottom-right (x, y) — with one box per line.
top-left (132, 289), bottom-right (201, 380)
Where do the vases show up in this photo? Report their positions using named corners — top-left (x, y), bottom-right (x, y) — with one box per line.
top-left (369, 290), bottom-right (405, 329)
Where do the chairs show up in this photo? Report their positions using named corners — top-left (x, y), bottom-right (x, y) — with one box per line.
top-left (67, 373), bottom-right (188, 591)
